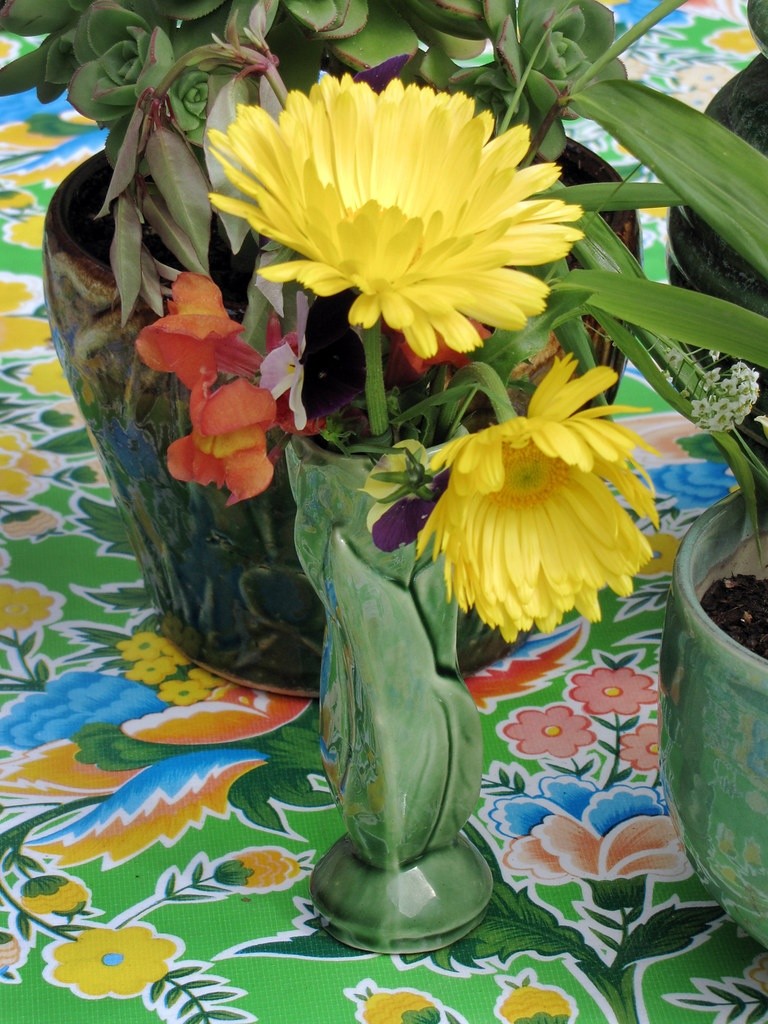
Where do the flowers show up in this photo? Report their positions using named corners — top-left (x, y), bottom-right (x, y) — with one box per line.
top-left (134, 72), bottom-right (759, 646)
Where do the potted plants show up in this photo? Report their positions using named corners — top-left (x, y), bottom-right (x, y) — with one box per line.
top-left (0, 0), bottom-right (644, 700)
top-left (528, 74), bottom-right (768, 953)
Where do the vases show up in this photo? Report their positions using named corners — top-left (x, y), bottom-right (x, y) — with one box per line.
top-left (667, 1), bottom-right (768, 466)
top-left (286, 415), bottom-right (496, 964)
